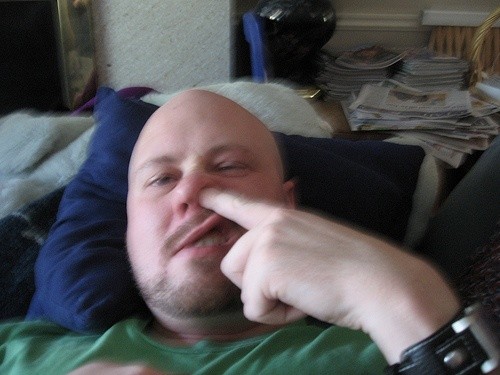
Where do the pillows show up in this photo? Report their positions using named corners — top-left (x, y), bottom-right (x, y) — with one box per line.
top-left (23, 86), bottom-right (424, 336)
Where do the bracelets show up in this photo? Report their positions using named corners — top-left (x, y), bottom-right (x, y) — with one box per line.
top-left (382, 291), bottom-right (500, 375)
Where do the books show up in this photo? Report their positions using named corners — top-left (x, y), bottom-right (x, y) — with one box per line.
top-left (291, 43), bottom-right (500, 167)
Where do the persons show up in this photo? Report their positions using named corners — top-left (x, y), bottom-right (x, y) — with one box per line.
top-left (1, 89), bottom-right (500, 375)
